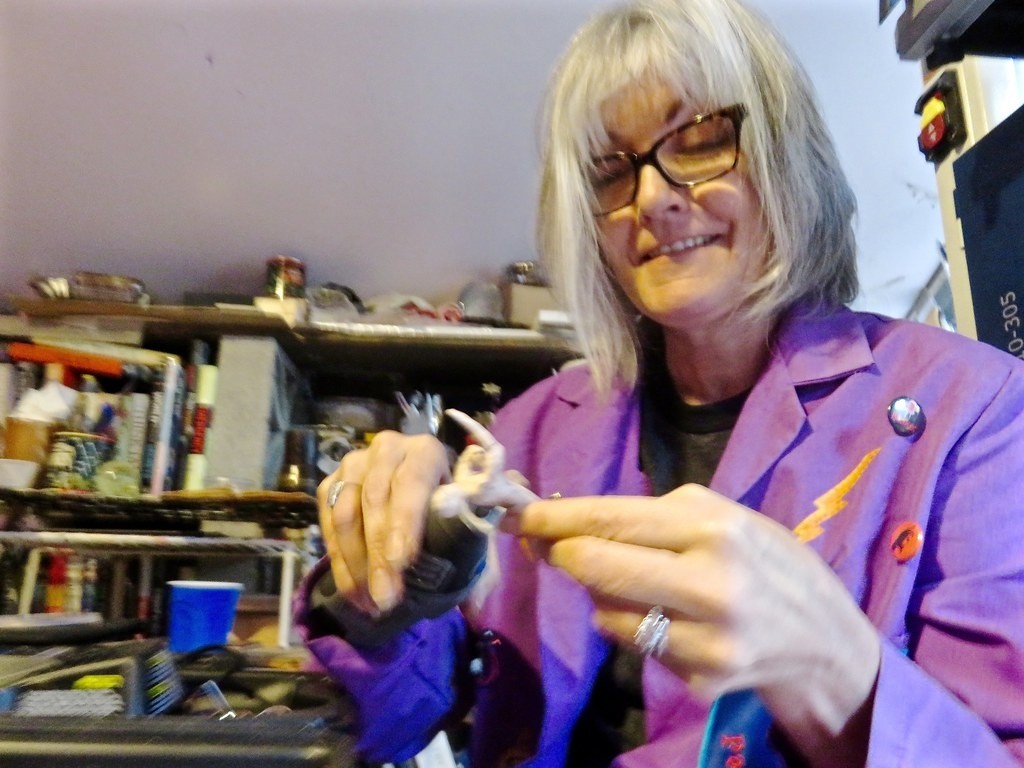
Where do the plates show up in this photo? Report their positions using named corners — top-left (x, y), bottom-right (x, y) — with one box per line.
top-left (28, 272), bottom-right (144, 303)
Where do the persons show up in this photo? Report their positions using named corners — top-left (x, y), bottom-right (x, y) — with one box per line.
top-left (317, 0), bottom-right (1024, 768)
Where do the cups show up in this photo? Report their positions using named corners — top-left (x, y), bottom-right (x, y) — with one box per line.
top-left (44, 428), bottom-right (118, 492)
top-left (5, 416), bottom-right (54, 463)
top-left (166, 580), bottom-right (243, 651)
top-left (0, 458), bottom-right (40, 489)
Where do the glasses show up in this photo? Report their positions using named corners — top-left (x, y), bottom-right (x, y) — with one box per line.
top-left (581, 102), bottom-right (750, 219)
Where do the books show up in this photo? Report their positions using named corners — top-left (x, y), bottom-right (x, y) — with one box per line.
top-left (127, 342), bottom-right (218, 491)
top-left (0, 549), bottom-right (194, 640)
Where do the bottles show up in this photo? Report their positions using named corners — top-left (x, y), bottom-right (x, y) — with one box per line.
top-left (303, 484), bottom-right (508, 651)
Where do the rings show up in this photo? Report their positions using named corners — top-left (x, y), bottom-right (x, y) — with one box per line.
top-left (326, 484), bottom-right (342, 502)
top-left (638, 612), bottom-right (667, 658)
